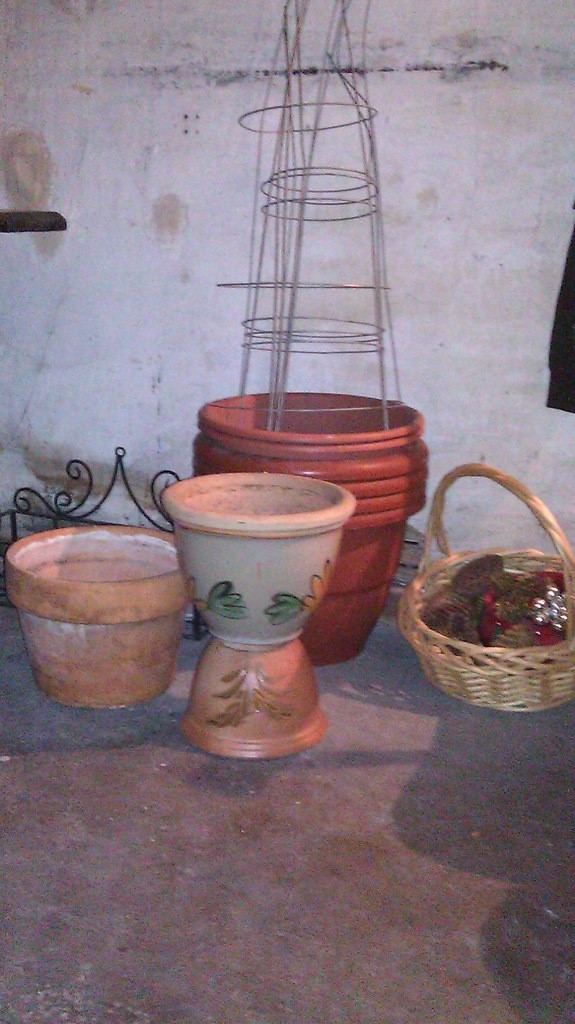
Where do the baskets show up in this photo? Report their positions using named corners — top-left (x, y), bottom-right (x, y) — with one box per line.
top-left (397, 463), bottom-right (575, 712)
